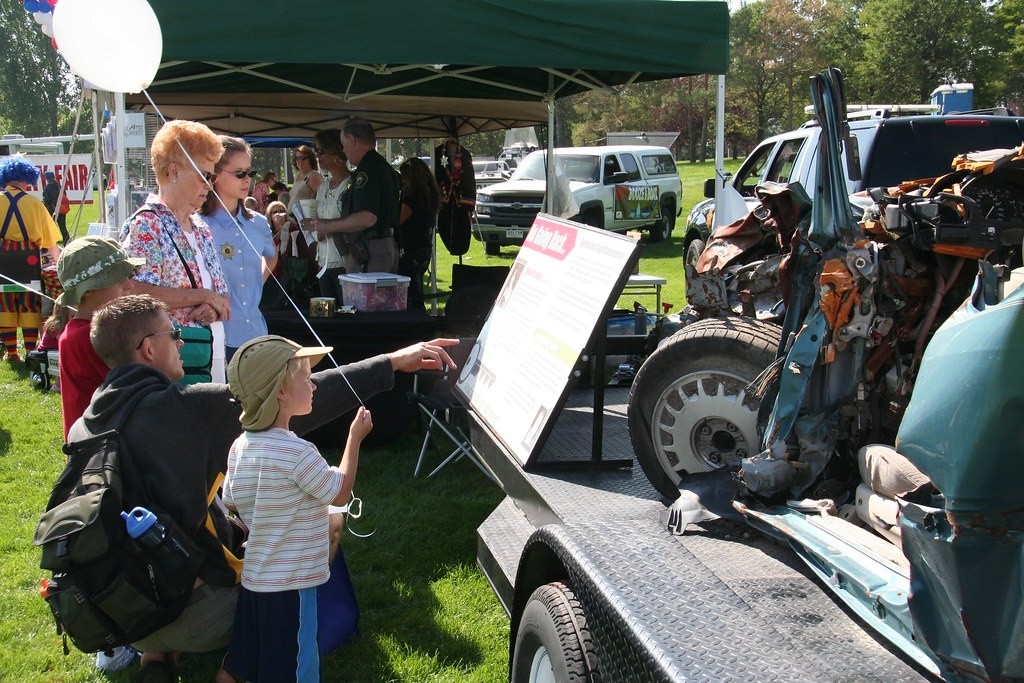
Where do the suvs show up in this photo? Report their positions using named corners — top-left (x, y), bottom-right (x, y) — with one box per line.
top-left (471, 152), bottom-right (529, 189)
top-left (683, 105), bottom-right (1024, 289)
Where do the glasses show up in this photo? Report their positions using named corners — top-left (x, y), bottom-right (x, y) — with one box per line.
top-left (136, 327), bottom-right (183, 350)
top-left (166, 167), bottom-right (218, 184)
top-left (314, 147), bottom-right (329, 155)
top-left (295, 154), bottom-right (313, 161)
top-left (270, 209), bottom-right (285, 215)
top-left (215, 166), bottom-right (257, 179)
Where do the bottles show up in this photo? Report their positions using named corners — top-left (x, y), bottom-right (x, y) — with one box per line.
top-left (121, 506), bottom-right (189, 564)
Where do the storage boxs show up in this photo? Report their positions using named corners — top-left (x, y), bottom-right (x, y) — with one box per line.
top-left (338, 271), bottom-right (411, 311)
top-left (930, 82), bottom-right (974, 116)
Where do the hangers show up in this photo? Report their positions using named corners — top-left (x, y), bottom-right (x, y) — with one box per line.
top-left (447, 128), bottom-right (459, 144)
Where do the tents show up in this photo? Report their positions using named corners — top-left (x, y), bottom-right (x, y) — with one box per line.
top-left (84, 0), bottom-right (730, 231)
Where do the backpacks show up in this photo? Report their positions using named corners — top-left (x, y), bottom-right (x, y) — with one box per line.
top-left (31, 384), bottom-right (209, 657)
top-left (50, 182), bottom-right (70, 214)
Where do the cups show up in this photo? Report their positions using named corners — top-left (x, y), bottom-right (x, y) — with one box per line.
top-left (299, 199), bottom-right (318, 219)
top-left (626, 230), bottom-right (639, 274)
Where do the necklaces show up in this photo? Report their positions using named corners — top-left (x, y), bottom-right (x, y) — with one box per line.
top-left (184, 223), bottom-right (197, 255)
top-left (327, 180), bottom-right (333, 197)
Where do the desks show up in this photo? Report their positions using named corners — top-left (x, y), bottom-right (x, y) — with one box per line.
top-left (626, 273), bottom-right (667, 324)
top-left (264, 310), bottom-right (441, 447)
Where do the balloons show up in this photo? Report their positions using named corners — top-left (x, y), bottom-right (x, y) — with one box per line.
top-left (21, 0), bottom-right (60, 48)
top-left (53, 0), bottom-right (163, 93)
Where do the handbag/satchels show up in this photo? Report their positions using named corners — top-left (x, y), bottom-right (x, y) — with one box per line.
top-left (172, 324), bottom-right (214, 388)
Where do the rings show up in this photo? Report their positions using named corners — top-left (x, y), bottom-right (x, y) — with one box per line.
top-left (202, 318), bottom-right (205, 322)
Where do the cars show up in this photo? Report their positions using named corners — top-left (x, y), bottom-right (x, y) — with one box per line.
top-left (92, 166), bottom-right (109, 190)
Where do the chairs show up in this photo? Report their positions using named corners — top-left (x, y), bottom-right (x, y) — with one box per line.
top-left (406, 335), bottom-right (495, 480)
top-left (429, 264), bottom-right (510, 339)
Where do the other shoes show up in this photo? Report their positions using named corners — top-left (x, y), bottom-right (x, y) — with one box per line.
top-left (136, 650), bottom-right (179, 683)
top-left (95, 643), bottom-right (143, 674)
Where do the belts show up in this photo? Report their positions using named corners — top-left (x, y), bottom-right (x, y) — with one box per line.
top-left (188, 582), bottom-right (222, 606)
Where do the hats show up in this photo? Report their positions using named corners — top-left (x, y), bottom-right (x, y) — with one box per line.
top-left (44, 171), bottom-right (55, 179)
top-left (56, 235), bottom-right (146, 306)
top-left (227, 335), bottom-right (334, 432)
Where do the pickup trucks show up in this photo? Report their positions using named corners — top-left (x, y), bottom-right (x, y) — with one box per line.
top-left (473, 146), bottom-right (683, 255)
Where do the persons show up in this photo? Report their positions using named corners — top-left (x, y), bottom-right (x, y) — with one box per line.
top-left (0, 118), bottom-right (460, 683)
